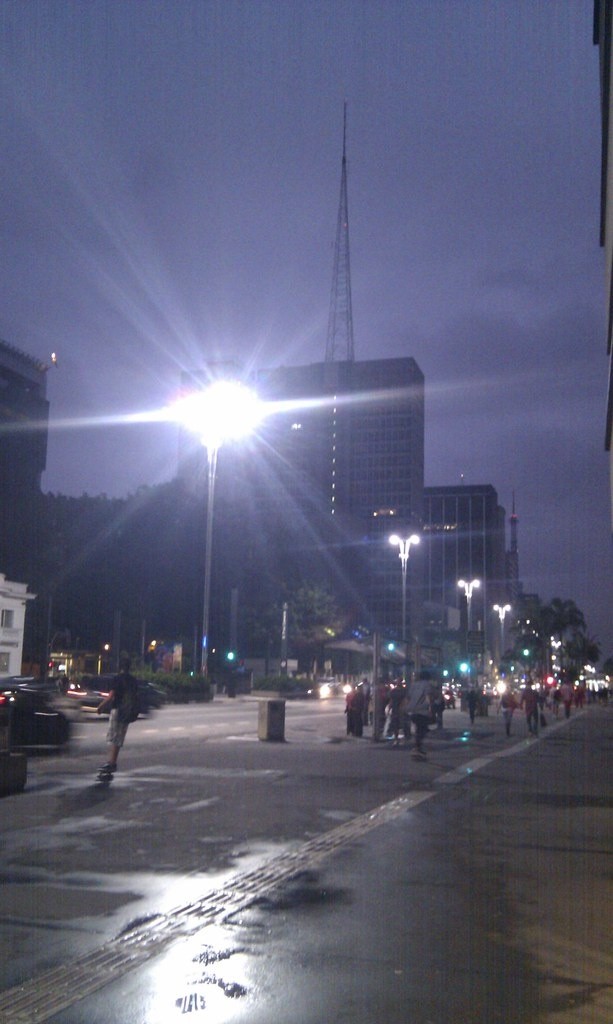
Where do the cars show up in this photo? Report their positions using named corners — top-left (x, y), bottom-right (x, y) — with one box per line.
top-left (0, 674), bottom-right (69, 798)
top-left (441, 686), bottom-right (456, 709)
top-left (68, 673), bottom-right (158, 723)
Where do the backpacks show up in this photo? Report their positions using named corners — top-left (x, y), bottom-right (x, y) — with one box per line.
top-left (117, 673), bottom-right (141, 722)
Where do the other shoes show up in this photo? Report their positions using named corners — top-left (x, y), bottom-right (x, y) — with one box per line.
top-left (97, 762), bottom-right (116, 772)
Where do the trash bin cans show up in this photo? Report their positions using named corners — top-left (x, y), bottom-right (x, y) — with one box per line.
top-left (257, 699), bottom-right (286, 741)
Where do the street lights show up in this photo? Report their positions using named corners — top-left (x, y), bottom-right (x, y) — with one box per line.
top-left (457, 579), bottom-right (481, 687)
top-left (389, 533), bottom-right (422, 682)
top-left (188, 380), bottom-right (264, 683)
top-left (493, 604), bottom-right (510, 658)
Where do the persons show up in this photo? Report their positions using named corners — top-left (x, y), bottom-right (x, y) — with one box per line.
top-left (97, 658), bottom-right (139, 772)
top-left (497, 682), bottom-right (608, 737)
top-left (466, 690), bottom-right (491, 721)
top-left (401, 671), bottom-right (439, 761)
top-left (345, 677), bottom-right (444, 747)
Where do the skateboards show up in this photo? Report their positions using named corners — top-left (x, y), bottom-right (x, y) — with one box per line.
top-left (97, 767), bottom-right (116, 781)
